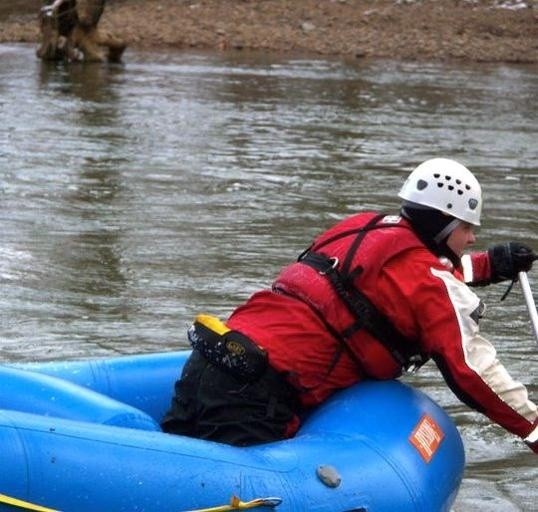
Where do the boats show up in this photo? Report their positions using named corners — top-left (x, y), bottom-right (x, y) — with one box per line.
top-left (0, 350), bottom-right (466, 512)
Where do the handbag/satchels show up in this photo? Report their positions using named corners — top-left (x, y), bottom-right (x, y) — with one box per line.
top-left (187, 313), bottom-right (268, 382)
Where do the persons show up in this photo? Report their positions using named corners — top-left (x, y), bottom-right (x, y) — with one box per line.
top-left (161, 157), bottom-right (538, 457)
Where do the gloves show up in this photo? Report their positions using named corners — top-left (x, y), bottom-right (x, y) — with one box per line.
top-left (489, 241), bottom-right (538, 284)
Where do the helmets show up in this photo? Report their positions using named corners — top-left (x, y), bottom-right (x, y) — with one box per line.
top-left (397, 158), bottom-right (482, 226)
